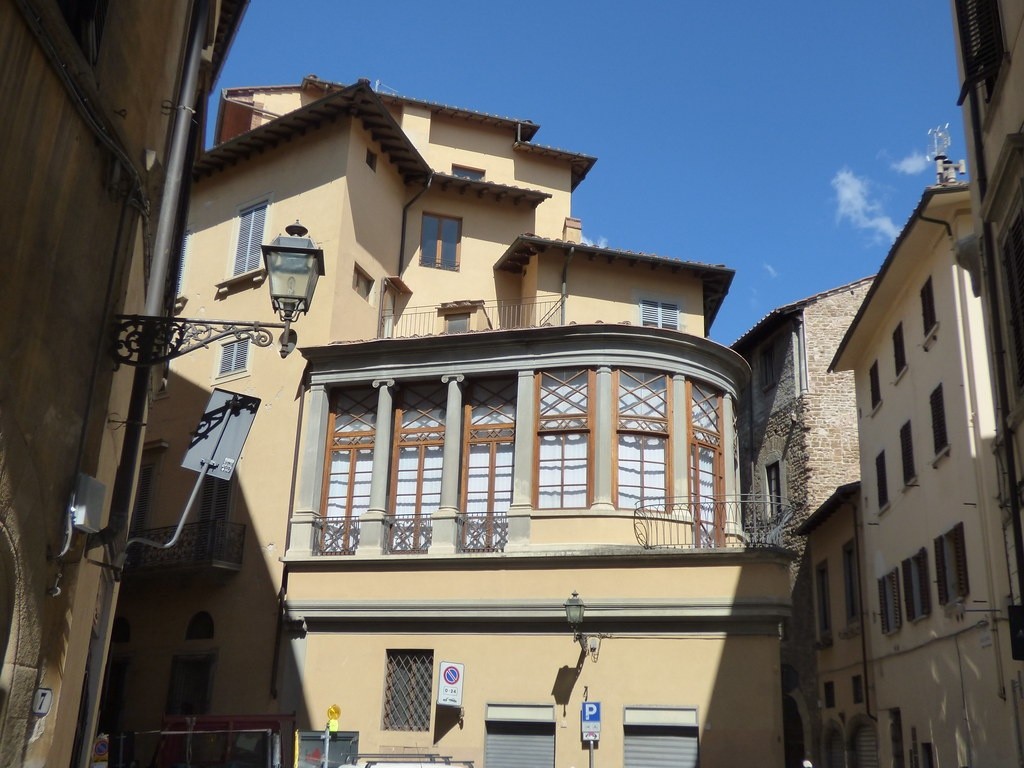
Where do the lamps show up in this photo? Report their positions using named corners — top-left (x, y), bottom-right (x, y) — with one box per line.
top-left (106, 219), bottom-right (326, 372)
top-left (563, 588), bottom-right (612, 663)
